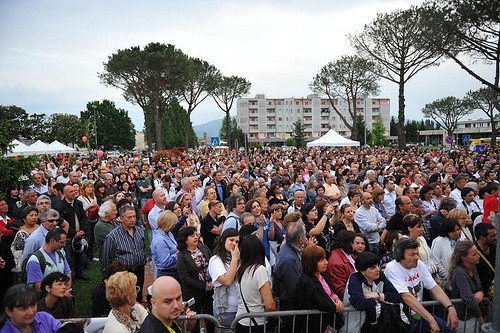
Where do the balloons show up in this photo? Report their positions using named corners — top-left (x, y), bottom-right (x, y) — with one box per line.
top-left (82, 137), bottom-right (87, 143)
top-left (97, 150), bottom-right (103, 157)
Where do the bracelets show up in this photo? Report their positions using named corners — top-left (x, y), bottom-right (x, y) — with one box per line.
top-left (447, 305), bottom-right (454, 311)
top-left (323, 213), bottom-right (330, 218)
top-left (376, 292), bottom-right (381, 301)
top-left (270, 221), bottom-right (274, 223)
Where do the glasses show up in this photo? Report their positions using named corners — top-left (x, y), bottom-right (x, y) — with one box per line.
top-left (136, 286), bottom-right (140, 294)
top-left (459, 217), bottom-right (466, 220)
top-left (53, 282), bottom-right (69, 286)
top-left (47, 218), bottom-right (61, 223)
top-left (416, 223), bottom-right (422, 229)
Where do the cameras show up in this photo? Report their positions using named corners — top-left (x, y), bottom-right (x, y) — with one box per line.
top-left (182, 297), bottom-right (195, 309)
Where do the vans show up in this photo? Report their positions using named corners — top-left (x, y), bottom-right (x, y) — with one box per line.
top-left (469, 137), bottom-right (500, 150)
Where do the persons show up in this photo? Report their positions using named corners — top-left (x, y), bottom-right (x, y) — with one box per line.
top-left (0, 144), bottom-right (500, 333)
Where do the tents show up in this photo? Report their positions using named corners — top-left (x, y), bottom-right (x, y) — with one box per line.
top-left (307, 129), bottom-right (360, 147)
top-left (7, 140), bottom-right (75, 153)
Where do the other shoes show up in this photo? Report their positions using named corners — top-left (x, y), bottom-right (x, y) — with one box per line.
top-left (87, 257), bottom-right (99, 263)
top-left (75, 273), bottom-right (91, 280)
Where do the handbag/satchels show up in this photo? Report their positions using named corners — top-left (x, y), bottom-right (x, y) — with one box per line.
top-left (88, 205), bottom-right (99, 220)
